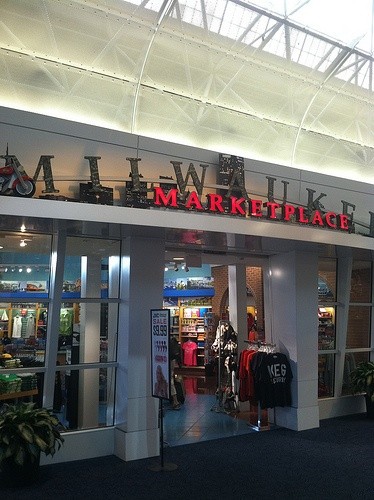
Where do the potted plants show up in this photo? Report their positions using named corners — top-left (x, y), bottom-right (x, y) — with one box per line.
top-left (0, 402), bottom-right (65, 488)
top-left (351, 361), bottom-right (374, 420)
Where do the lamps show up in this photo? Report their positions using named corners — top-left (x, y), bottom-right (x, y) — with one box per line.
top-left (174, 264), bottom-right (179, 271)
top-left (185, 267), bottom-right (189, 272)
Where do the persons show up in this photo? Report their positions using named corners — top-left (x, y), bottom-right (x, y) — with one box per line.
top-left (170, 335), bottom-right (180, 410)
top-left (248, 323), bottom-right (259, 342)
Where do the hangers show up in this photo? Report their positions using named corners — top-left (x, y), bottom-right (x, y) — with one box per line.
top-left (247, 341), bottom-right (278, 354)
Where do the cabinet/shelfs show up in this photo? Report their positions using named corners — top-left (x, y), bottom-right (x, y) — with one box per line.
top-left (0, 295), bottom-right (213, 437)
